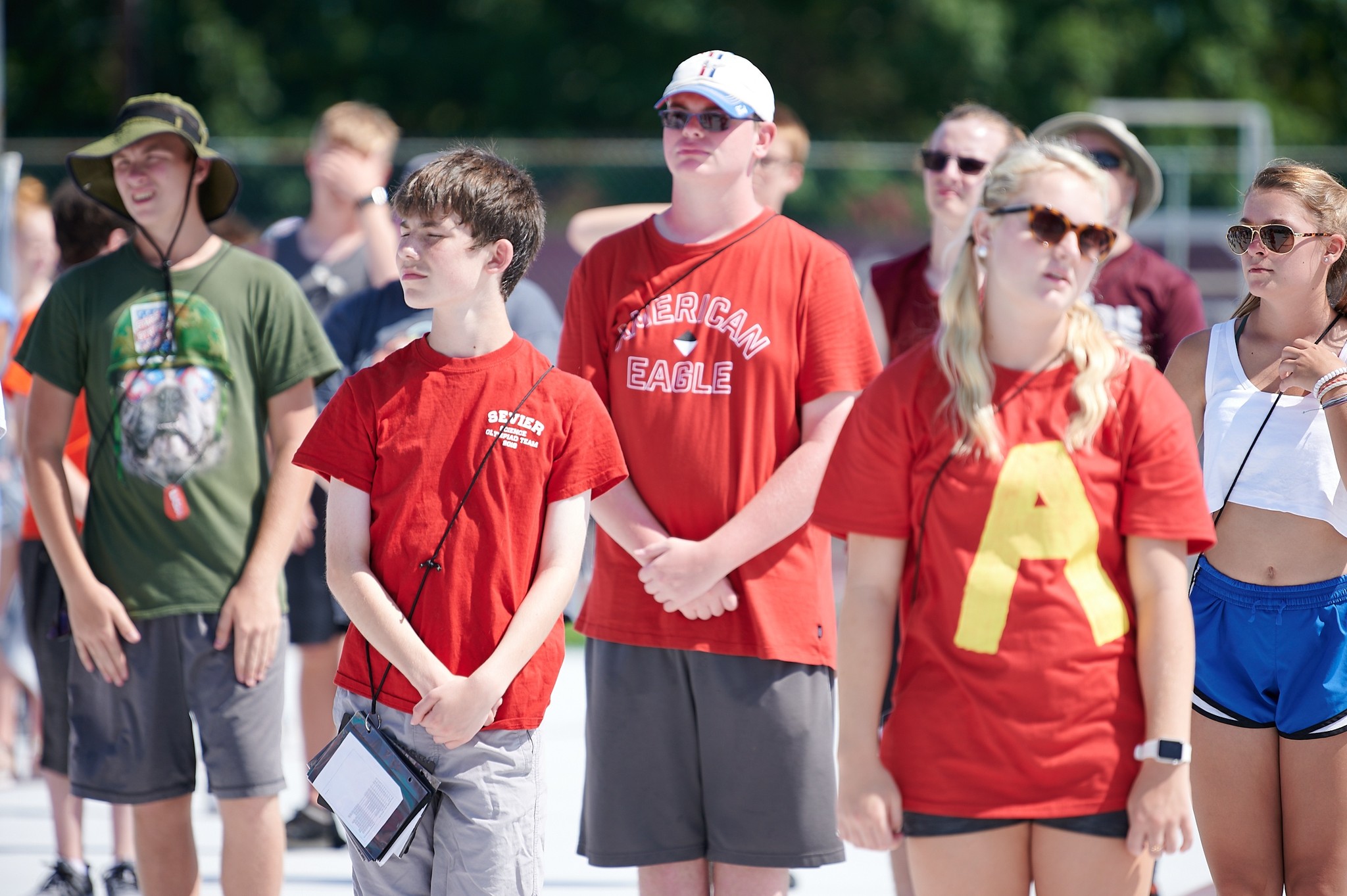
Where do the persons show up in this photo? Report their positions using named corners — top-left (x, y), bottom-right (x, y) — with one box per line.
top-left (1161, 153), bottom-right (1347, 896)
top-left (2, 51), bottom-right (1216, 895)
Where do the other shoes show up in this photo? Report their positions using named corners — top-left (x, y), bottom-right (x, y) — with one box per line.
top-left (286, 805), bottom-right (347, 848)
top-left (38, 859), bottom-right (93, 896)
top-left (102, 862), bottom-right (139, 896)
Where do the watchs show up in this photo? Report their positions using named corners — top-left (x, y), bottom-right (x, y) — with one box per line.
top-left (352, 183), bottom-right (389, 210)
top-left (1133, 738), bottom-right (1193, 767)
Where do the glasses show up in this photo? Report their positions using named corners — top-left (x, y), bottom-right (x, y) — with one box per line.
top-left (988, 201), bottom-right (1120, 264)
top-left (920, 149), bottom-right (986, 176)
top-left (658, 109), bottom-right (763, 132)
top-left (1091, 150), bottom-right (1123, 171)
top-left (1226, 224), bottom-right (1332, 255)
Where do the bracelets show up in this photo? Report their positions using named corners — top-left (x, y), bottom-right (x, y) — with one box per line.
top-left (1303, 367), bottom-right (1347, 413)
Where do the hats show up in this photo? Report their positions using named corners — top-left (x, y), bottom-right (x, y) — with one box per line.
top-left (654, 50), bottom-right (775, 122)
top-left (65, 93), bottom-right (238, 224)
top-left (1031, 111), bottom-right (1164, 230)
